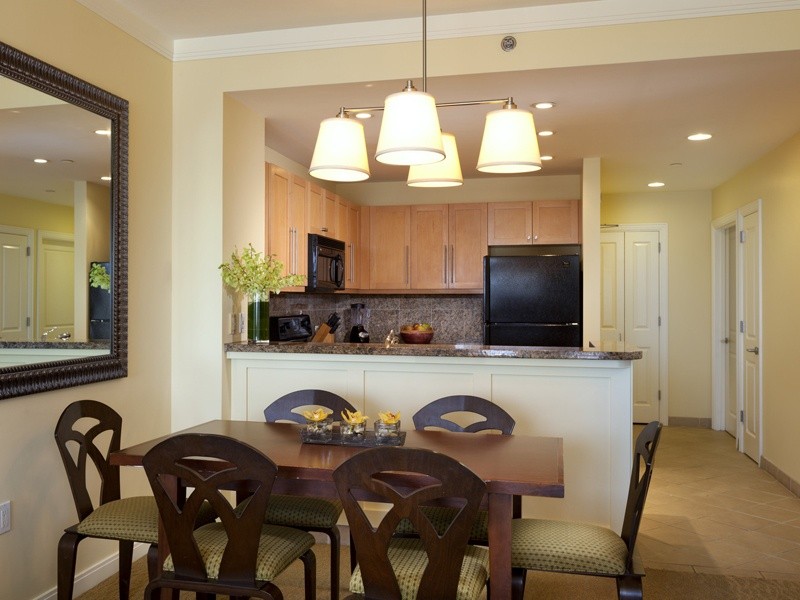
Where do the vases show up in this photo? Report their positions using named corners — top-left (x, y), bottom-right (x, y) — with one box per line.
top-left (304, 417), bottom-right (333, 443)
top-left (339, 420), bottom-right (367, 445)
top-left (245, 288), bottom-right (270, 345)
top-left (374, 419), bottom-right (401, 446)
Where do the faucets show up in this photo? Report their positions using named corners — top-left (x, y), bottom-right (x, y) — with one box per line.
top-left (41, 326), bottom-right (71, 342)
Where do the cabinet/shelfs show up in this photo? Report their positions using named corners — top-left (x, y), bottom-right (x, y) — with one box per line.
top-left (263, 157), bottom-right (583, 295)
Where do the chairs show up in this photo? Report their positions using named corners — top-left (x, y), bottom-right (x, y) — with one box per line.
top-left (54, 399), bottom-right (218, 600)
top-left (332, 445), bottom-right (490, 600)
top-left (392, 394), bottom-right (516, 547)
top-left (233, 389), bottom-right (357, 600)
top-left (511, 420), bottom-right (664, 600)
top-left (141, 432), bottom-right (318, 600)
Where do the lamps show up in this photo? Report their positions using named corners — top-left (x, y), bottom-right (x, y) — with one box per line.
top-left (307, 0), bottom-right (544, 190)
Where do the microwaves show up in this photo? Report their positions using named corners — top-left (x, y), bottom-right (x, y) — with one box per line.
top-left (305, 234), bottom-right (346, 292)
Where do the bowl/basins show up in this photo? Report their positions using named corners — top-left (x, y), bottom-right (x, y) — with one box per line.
top-left (401, 331), bottom-right (433, 344)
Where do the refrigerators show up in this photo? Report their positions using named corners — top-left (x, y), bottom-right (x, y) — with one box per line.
top-left (483, 254), bottom-right (583, 347)
top-left (89, 261), bottom-right (111, 339)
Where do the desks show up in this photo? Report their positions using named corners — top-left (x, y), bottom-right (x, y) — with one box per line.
top-left (109, 419), bottom-right (565, 600)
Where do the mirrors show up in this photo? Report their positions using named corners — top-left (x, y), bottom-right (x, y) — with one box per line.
top-left (0, 42), bottom-right (129, 401)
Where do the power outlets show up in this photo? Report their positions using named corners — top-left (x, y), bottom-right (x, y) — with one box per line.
top-left (0, 501), bottom-right (12, 535)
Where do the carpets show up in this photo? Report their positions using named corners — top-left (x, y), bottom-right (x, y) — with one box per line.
top-left (74, 544), bottom-right (800, 600)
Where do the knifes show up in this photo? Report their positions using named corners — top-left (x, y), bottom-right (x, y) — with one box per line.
top-left (327, 313), bottom-right (341, 336)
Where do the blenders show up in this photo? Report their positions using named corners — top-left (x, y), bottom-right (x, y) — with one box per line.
top-left (350, 303), bottom-right (369, 343)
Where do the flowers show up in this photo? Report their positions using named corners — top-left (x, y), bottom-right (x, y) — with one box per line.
top-left (302, 407), bottom-right (333, 428)
top-left (380, 412), bottom-right (400, 432)
top-left (339, 406), bottom-right (369, 433)
top-left (219, 241), bottom-right (306, 302)
top-left (88, 263), bottom-right (112, 293)
